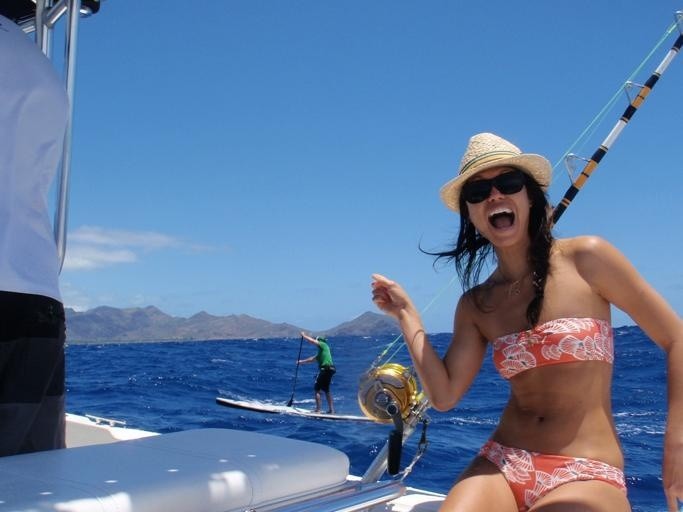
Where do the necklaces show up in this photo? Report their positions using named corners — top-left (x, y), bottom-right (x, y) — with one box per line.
top-left (498, 266), bottom-right (533, 296)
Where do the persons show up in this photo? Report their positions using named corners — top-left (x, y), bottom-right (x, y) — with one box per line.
top-left (371, 132), bottom-right (683, 511)
top-left (0, 12), bottom-right (72, 458)
top-left (296, 331), bottom-right (337, 414)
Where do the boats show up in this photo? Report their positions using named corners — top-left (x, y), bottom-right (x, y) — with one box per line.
top-left (216, 397), bottom-right (378, 423)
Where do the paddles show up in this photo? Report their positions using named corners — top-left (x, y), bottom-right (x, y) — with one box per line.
top-left (287, 335), bottom-right (304, 407)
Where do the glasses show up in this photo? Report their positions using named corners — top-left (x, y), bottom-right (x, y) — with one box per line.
top-left (460, 171), bottom-right (524, 204)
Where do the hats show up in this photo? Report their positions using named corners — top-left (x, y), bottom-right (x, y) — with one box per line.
top-left (438, 132), bottom-right (552, 214)
